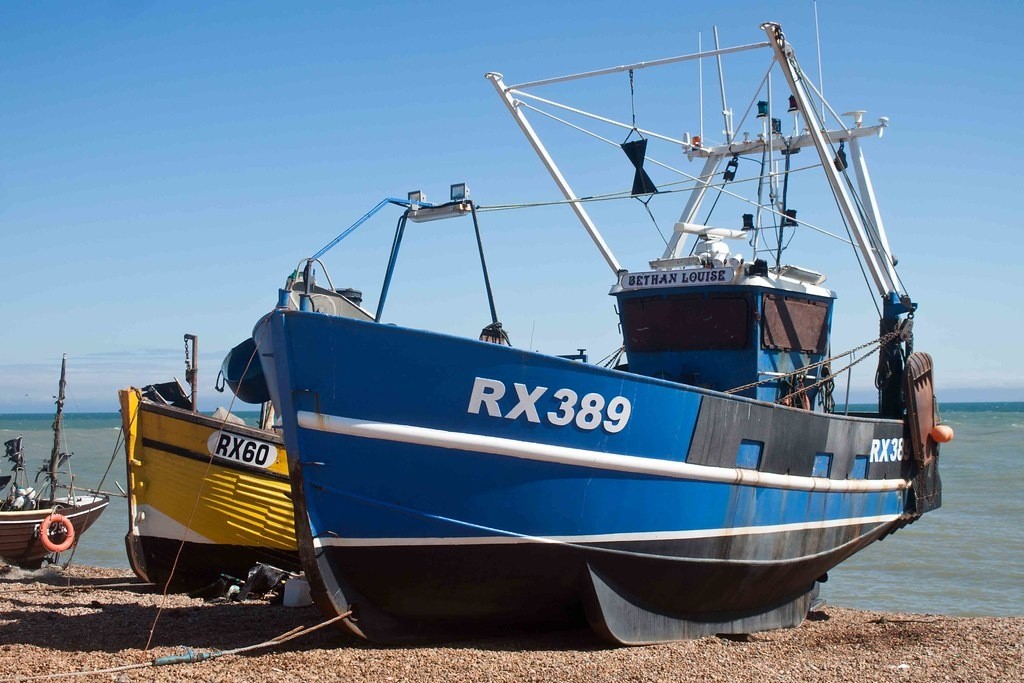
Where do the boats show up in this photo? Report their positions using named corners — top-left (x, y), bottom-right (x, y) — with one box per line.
top-left (1, 349), bottom-right (116, 569)
top-left (218, 0), bottom-right (953, 647)
top-left (120, 332), bottom-right (304, 597)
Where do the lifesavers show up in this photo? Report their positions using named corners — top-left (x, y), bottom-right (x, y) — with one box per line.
top-left (39, 513), bottom-right (76, 553)
